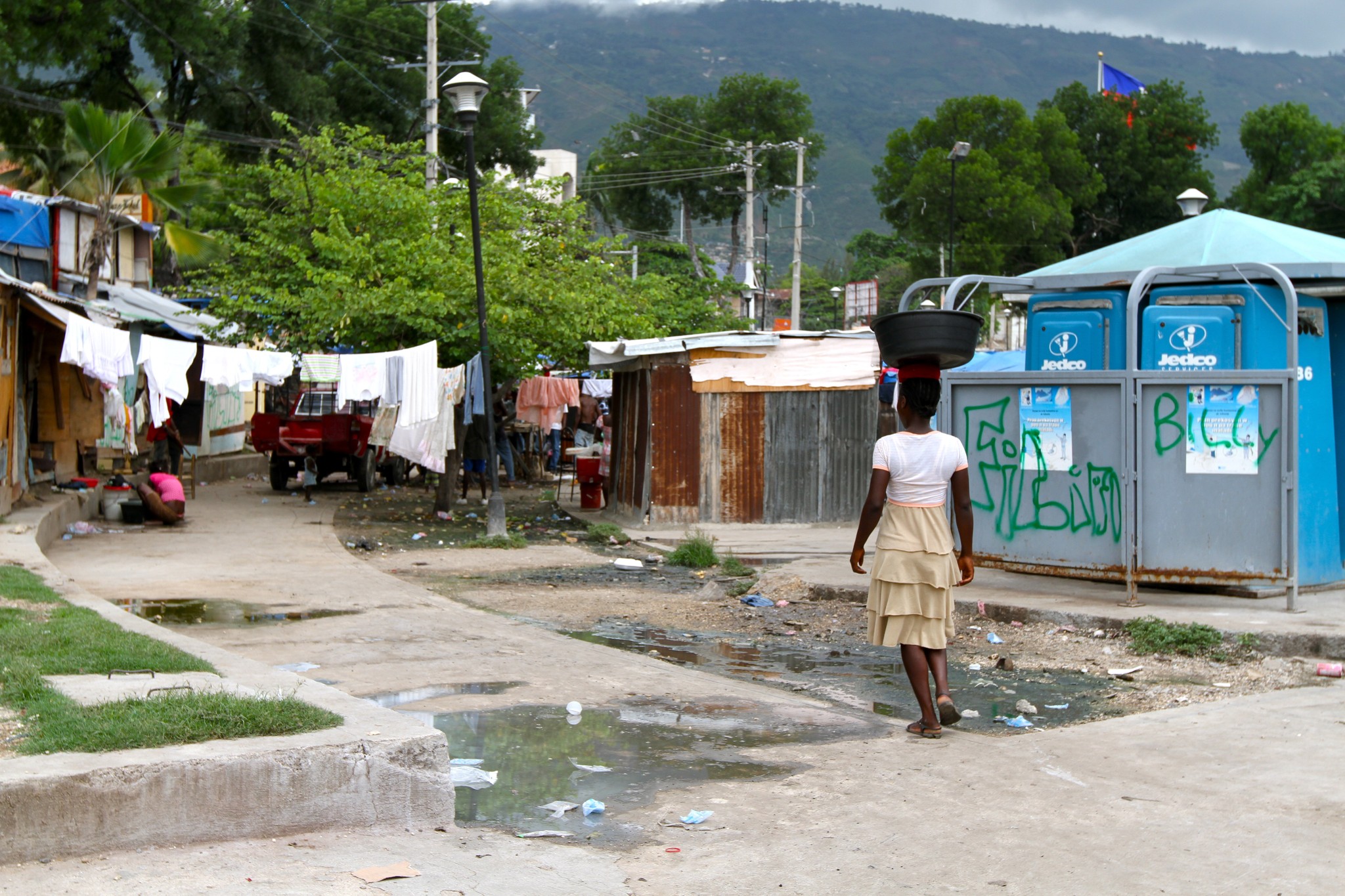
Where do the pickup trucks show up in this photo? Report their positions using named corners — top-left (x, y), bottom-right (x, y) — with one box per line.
top-left (251, 378), bottom-right (410, 493)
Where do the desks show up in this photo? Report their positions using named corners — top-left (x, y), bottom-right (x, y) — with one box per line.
top-left (504, 422), bottom-right (543, 484)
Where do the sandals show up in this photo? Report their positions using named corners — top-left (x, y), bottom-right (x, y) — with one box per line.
top-left (906, 721), bottom-right (942, 739)
top-left (936, 693), bottom-right (962, 726)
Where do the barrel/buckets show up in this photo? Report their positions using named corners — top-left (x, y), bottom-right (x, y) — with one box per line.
top-left (121, 501), bottom-right (145, 524)
top-left (102, 489), bottom-right (129, 520)
top-left (580, 483), bottom-right (602, 509)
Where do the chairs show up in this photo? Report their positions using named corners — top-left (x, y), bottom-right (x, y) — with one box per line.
top-left (557, 427), bottom-right (578, 501)
top-left (176, 454), bottom-right (195, 499)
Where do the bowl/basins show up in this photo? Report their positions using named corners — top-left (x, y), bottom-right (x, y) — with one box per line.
top-left (71, 477), bottom-right (99, 488)
top-left (869, 309), bottom-right (986, 370)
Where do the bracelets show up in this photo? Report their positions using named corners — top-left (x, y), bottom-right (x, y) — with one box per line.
top-left (175, 429), bottom-right (179, 432)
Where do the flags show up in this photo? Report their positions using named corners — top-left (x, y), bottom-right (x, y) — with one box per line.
top-left (1100, 61), bottom-right (1148, 98)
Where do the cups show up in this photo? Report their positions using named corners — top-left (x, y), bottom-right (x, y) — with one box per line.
top-left (566, 701), bottom-right (582, 715)
top-left (566, 713), bottom-right (582, 725)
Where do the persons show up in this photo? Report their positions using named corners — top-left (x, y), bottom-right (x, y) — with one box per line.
top-left (137, 459), bottom-right (186, 526)
top-left (544, 404), bottom-right (569, 473)
top-left (572, 386), bottom-right (602, 476)
top-left (456, 394), bottom-right (490, 506)
top-left (491, 386), bottom-right (517, 487)
top-left (154, 411), bottom-right (181, 475)
top-left (592, 395), bottom-right (613, 509)
top-left (302, 443), bottom-right (320, 505)
top-left (849, 377), bottom-right (975, 738)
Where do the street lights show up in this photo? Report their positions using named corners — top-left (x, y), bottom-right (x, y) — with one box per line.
top-left (442, 71), bottom-right (513, 539)
top-left (948, 140), bottom-right (971, 278)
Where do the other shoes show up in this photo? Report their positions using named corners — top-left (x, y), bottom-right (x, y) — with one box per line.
top-left (456, 497), bottom-right (466, 504)
top-left (480, 497), bottom-right (488, 505)
top-left (508, 481), bottom-right (516, 486)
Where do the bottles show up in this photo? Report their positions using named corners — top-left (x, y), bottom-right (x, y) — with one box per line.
top-left (438, 511), bottom-right (452, 519)
